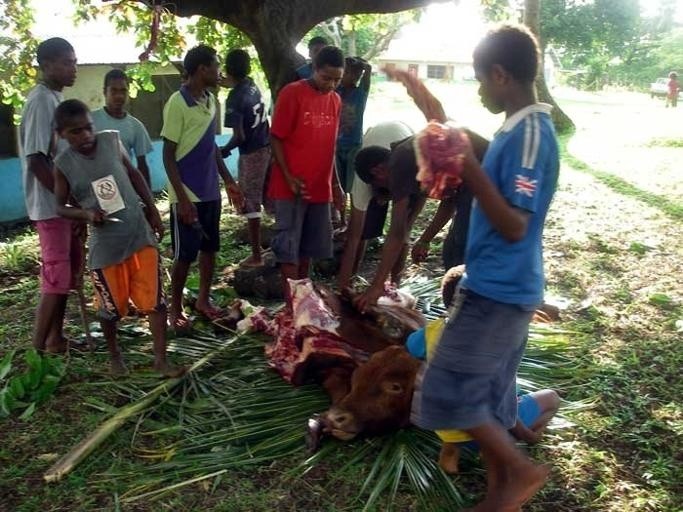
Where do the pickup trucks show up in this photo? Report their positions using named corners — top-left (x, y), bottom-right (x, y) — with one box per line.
top-left (649, 78), bottom-right (681, 98)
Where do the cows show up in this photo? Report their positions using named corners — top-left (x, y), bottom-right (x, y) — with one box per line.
top-left (227, 276), bottom-right (428, 443)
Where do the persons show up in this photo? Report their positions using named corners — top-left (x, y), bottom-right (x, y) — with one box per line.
top-left (404, 261), bottom-right (559, 475)
top-left (89, 66), bottom-right (155, 315)
top-left (259, 34), bottom-right (497, 312)
top-left (408, 22), bottom-right (561, 510)
top-left (664, 70), bottom-right (681, 108)
top-left (50, 95), bottom-right (193, 384)
top-left (15, 36), bottom-right (97, 362)
top-left (158, 44), bottom-right (226, 334)
top-left (214, 45), bottom-right (273, 268)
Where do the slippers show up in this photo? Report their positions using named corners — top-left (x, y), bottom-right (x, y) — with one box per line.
top-left (35, 339), bottom-right (96, 356)
top-left (169, 315), bottom-right (193, 330)
top-left (193, 301), bottom-right (228, 321)
